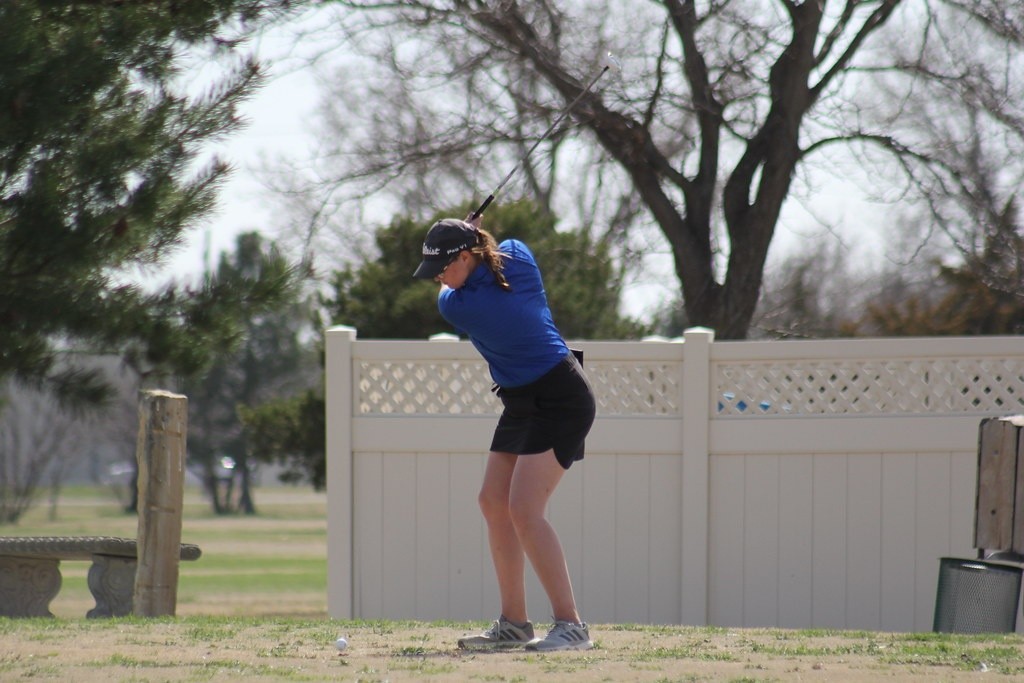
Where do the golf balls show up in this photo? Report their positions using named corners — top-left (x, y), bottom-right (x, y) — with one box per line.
top-left (336, 638), bottom-right (347, 651)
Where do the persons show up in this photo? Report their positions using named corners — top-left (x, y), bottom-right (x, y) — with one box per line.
top-left (412, 210), bottom-right (600, 653)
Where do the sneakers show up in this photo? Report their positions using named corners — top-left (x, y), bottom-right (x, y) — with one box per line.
top-left (526, 620), bottom-right (594, 651)
top-left (457, 615), bottom-right (534, 648)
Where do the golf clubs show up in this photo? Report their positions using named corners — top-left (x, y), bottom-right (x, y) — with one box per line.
top-left (471, 51), bottom-right (622, 219)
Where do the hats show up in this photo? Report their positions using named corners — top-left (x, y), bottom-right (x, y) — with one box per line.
top-left (410, 218), bottom-right (477, 278)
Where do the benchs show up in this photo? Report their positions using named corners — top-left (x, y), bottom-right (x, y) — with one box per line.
top-left (0, 536), bottom-right (201, 622)
